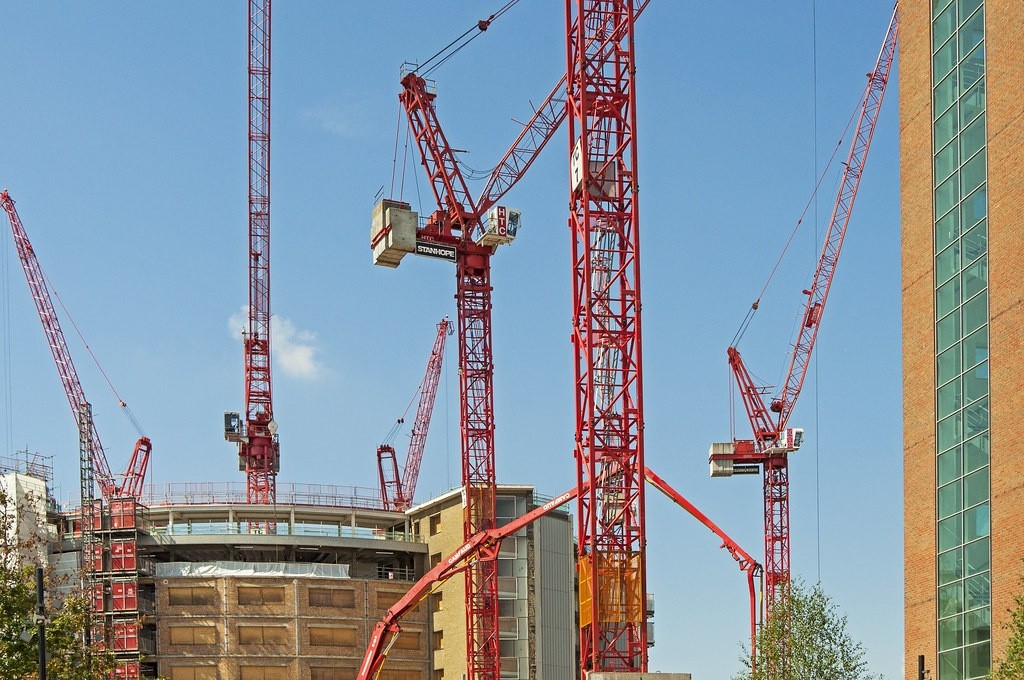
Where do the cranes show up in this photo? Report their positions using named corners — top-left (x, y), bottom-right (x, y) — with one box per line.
top-left (710, 2), bottom-right (907, 678)
top-left (368, 0), bottom-right (770, 680)
top-left (216, 0), bottom-right (312, 540)
top-left (1, 185), bottom-right (160, 678)
